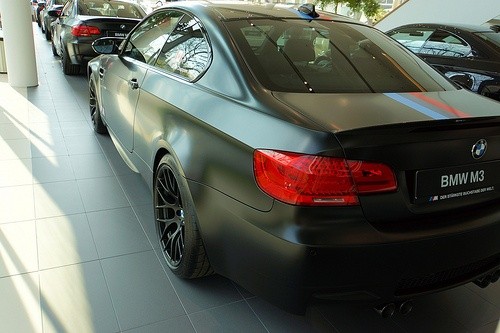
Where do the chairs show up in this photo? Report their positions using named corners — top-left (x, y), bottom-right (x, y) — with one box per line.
top-left (429, 39), bottom-right (447, 55)
top-left (117, 9), bottom-right (128, 17)
top-left (278, 38), bottom-right (335, 89)
top-left (88, 9), bottom-right (102, 16)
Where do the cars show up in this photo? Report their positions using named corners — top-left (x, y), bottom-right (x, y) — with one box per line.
top-left (31, 0), bottom-right (71, 40)
top-left (357, 21), bottom-right (500, 105)
top-left (88, 0), bottom-right (500, 316)
top-left (48, 0), bottom-right (150, 75)
